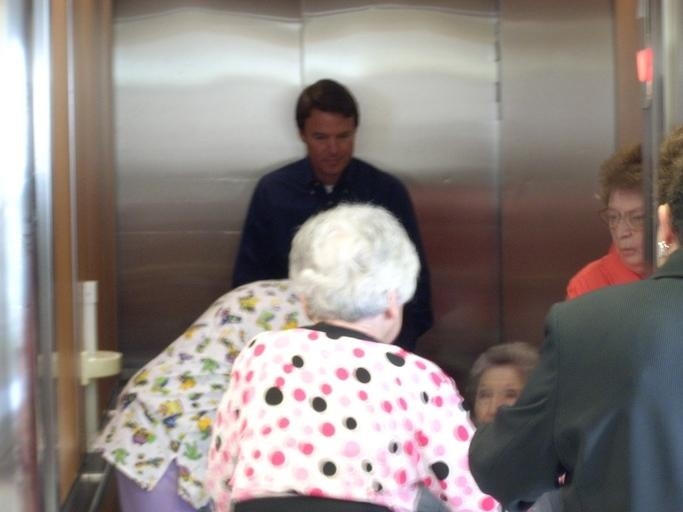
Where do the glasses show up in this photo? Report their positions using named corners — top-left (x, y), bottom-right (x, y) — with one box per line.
top-left (597, 204), bottom-right (645, 231)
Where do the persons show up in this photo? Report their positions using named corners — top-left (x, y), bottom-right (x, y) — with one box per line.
top-left (564, 143), bottom-right (660, 298)
top-left (89, 213), bottom-right (314, 512)
top-left (206, 201), bottom-right (502, 510)
top-left (468, 127), bottom-right (681, 512)
top-left (463, 341), bottom-right (539, 426)
top-left (231, 77), bottom-right (435, 351)
